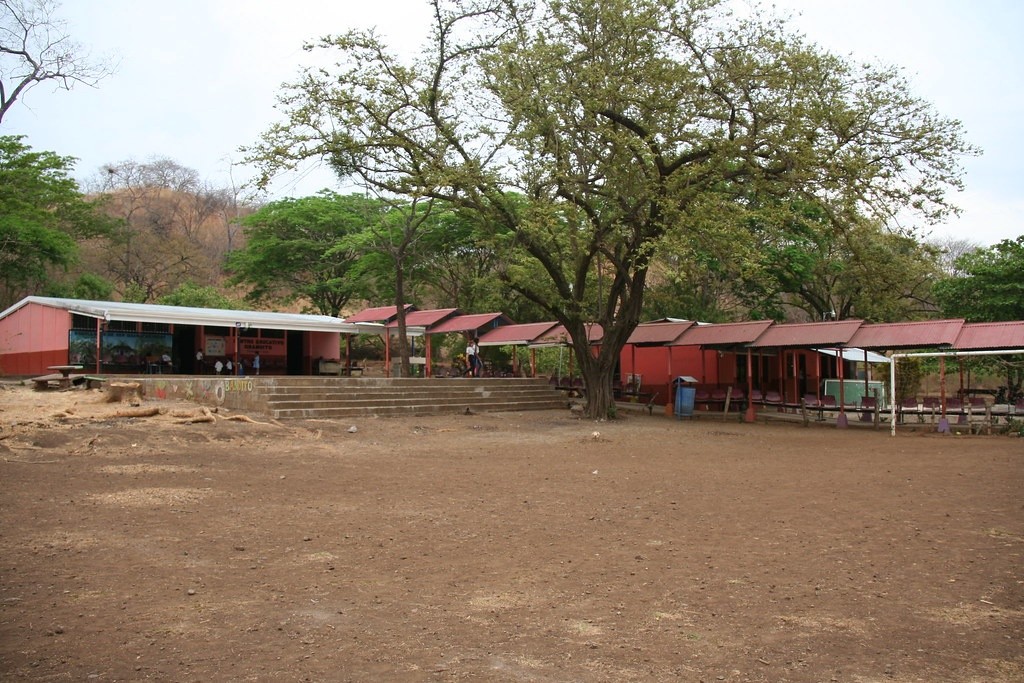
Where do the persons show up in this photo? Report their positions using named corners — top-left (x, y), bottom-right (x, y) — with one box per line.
top-left (215, 358), bottom-right (223, 374)
top-left (460, 337), bottom-right (482, 377)
top-left (194, 348), bottom-right (207, 375)
top-left (137, 352), bottom-right (155, 376)
top-left (239, 356), bottom-right (248, 374)
top-left (225, 359), bottom-right (233, 374)
top-left (158, 351), bottom-right (175, 373)
top-left (252, 351), bottom-right (263, 376)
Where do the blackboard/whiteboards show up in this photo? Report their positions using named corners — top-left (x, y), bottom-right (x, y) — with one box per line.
top-left (824, 379), bottom-right (885, 408)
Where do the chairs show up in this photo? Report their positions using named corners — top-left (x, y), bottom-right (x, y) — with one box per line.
top-left (146, 357), bottom-right (161, 374)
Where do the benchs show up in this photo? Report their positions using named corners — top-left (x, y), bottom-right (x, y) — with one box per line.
top-left (31, 374), bottom-right (106, 389)
top-left (549, 377), bottom-right (622, 399)
top-left (695, 388), bottom-right (1024, 425)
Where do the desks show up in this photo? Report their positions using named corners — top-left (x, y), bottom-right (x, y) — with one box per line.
top-left (47, 366), bottom-right (83, 376)
top-left (340, 367), bottom-right (363, 376)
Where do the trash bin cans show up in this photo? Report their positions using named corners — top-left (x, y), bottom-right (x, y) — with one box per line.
top-left (672, 376), bottom-right (699, 417)
